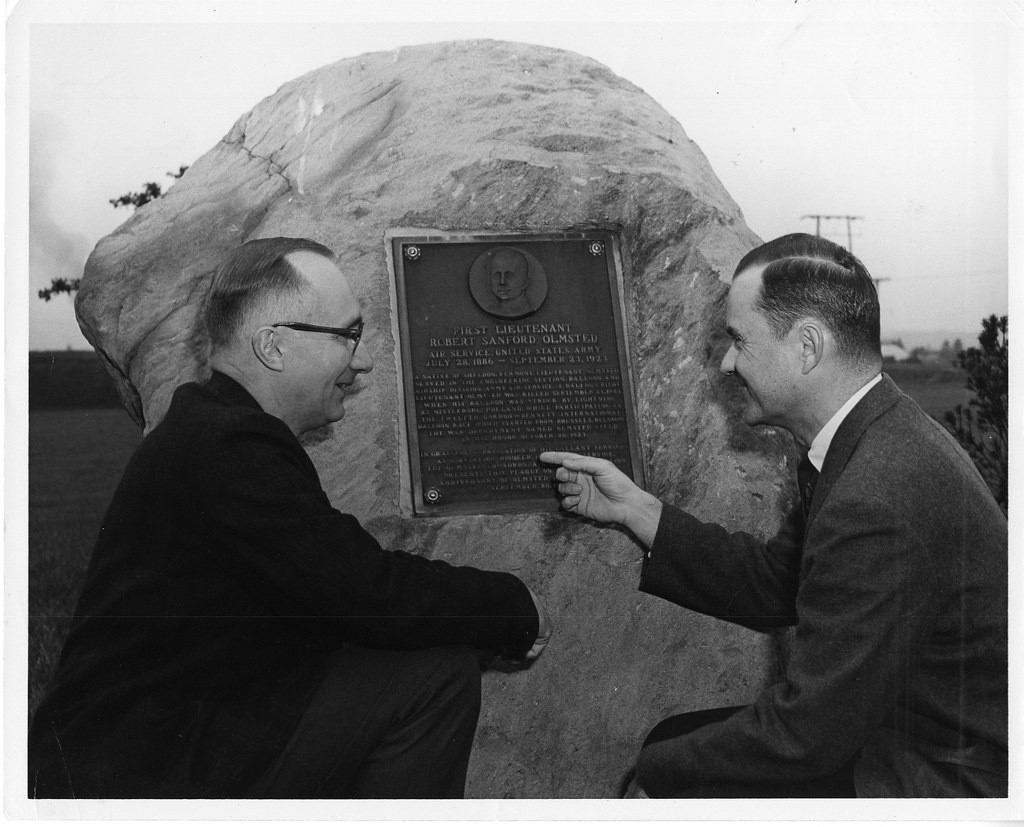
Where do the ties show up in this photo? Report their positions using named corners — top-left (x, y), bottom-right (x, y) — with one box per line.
top-left (797, 458), bottom-right (818, 524)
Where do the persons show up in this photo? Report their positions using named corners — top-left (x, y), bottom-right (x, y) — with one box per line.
top-left (539, 234), bottom-right (1008, 797)
top-left (29, 237), bottom-right (551, 799)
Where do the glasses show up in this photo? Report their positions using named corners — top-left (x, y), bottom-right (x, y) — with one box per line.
top-left (272, 320), bottom-right (365, 343)
